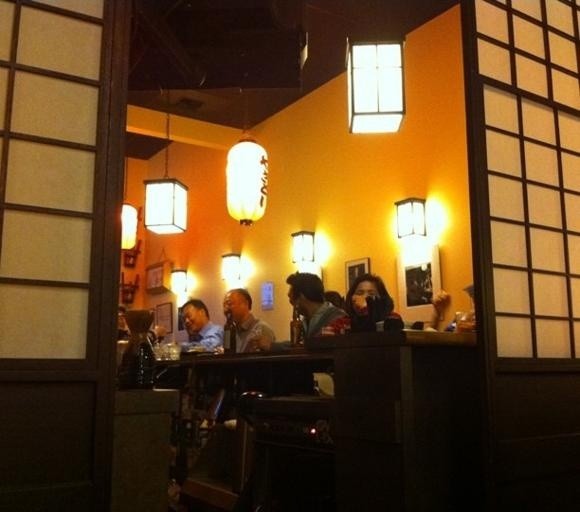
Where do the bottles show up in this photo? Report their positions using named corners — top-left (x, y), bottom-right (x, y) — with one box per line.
top-left (222, 309), bottom-right (237, 355)
top-left (290, 307), bottom-right (304, 344)
top-left (118, 308), bottom-right (159, 390)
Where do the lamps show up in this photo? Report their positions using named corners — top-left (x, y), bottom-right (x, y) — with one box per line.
top-left (226, 88), bottom-right (270, 223)
top-left (395, 198), bottom-right (426, 238)
top-left (345, 36), bottom-right (405, 133)
top-left (292, 232), bottom-right (313, 263)
top-left (142, 92), bottom-right (187, 234)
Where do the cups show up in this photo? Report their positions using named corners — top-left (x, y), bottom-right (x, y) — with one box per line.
top-left (154, 343), bottom-right (182, 360)
top-left (454, 311), bottom-right (472, 334)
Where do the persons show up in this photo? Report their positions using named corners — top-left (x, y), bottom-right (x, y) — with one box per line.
top-left (118, 306), bottom-right (130, 340)
top-left (221, 289), bottom-right (276, 418)
top-left (287, 272), bottom-right (404, 442)
top-left (180, 300), bottom-right (224, 412)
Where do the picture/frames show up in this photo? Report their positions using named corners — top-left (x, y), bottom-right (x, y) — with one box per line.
top-left (156, 302), bottom-right (173, 334)
top-left (397, 244), bottom-right (442, 322)
top-left (345, 257), bottom-right (370, 295)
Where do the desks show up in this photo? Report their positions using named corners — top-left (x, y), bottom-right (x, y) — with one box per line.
top-left (110, 388), bottom-right (180, 512)
top-left (306, 333), bottom-right (476, 508)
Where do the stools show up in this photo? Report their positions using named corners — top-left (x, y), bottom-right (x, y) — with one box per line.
top-left (234, 390), bottom-right (333, 494)
